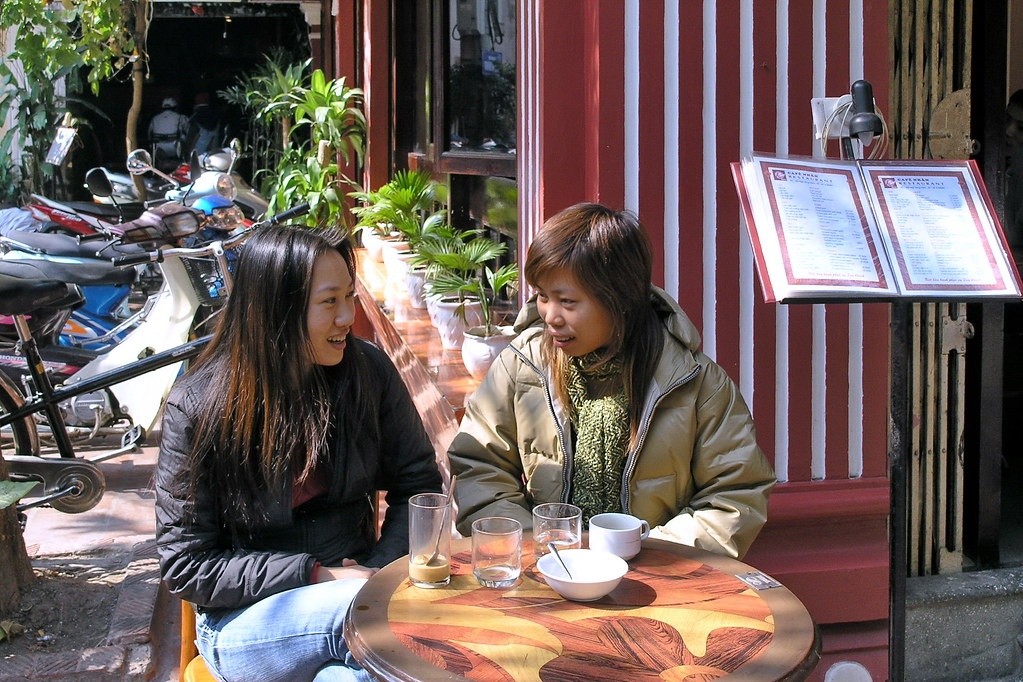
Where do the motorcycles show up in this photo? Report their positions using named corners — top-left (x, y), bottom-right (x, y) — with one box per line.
top-left (2, 124), bottom-right (274, 451)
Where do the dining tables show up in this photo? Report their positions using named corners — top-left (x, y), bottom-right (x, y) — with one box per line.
top-left (341, 524), bottom-right (822, 682)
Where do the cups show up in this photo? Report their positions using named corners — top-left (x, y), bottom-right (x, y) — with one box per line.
top-left (588, 512), bottom-right (649, 559)
top-left (472, 515), bottom-right (523, 589)
top-left (409, 493), bottom-right (454, 590)
top-left (532, 502), bottom-right (582, 559)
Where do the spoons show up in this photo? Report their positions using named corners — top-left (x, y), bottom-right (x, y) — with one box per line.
top-left (548, 543), bottom-right (572, 580)
top-left (426, 475), bottom-right (459, 565)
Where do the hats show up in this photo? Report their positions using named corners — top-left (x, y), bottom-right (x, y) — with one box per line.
top-left (194, 93), bottom-right (212, 105)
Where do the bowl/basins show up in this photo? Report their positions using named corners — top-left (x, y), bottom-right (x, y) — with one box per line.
top-left (534, 549), bottom-right (629, 602)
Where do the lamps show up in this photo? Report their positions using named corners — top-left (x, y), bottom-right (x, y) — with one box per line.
top-left (811, 79), bottom-right (884, 147)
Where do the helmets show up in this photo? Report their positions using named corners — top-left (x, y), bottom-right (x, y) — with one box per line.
top-left (162, 97), bottom-right (177, 108)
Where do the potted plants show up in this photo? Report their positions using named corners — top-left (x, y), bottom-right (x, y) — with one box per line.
top-left (349, 166), bottom-right (518, 384)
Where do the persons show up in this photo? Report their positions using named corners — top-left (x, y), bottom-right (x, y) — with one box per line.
top-left (445, 203), bottom-right (777, 562)
top-left (999, 87), bottom-right (1023, 480)
top-left (139, 78), bottom-right (240, 190)
top-left (154, 219), bottom-right (441, 682)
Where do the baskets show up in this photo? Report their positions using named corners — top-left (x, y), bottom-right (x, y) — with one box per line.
top-left (179, 242), bottom-right (246, 308)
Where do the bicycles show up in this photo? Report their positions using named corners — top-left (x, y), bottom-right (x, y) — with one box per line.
top-left (0, 202), bottom-right (313, 534)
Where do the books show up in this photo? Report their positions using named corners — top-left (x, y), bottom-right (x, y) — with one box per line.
top-left (728, 151), bottom-right (1022, 305)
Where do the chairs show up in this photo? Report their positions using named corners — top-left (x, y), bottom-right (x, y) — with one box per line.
top-left (177, 598), bottom-right (221, 681)
